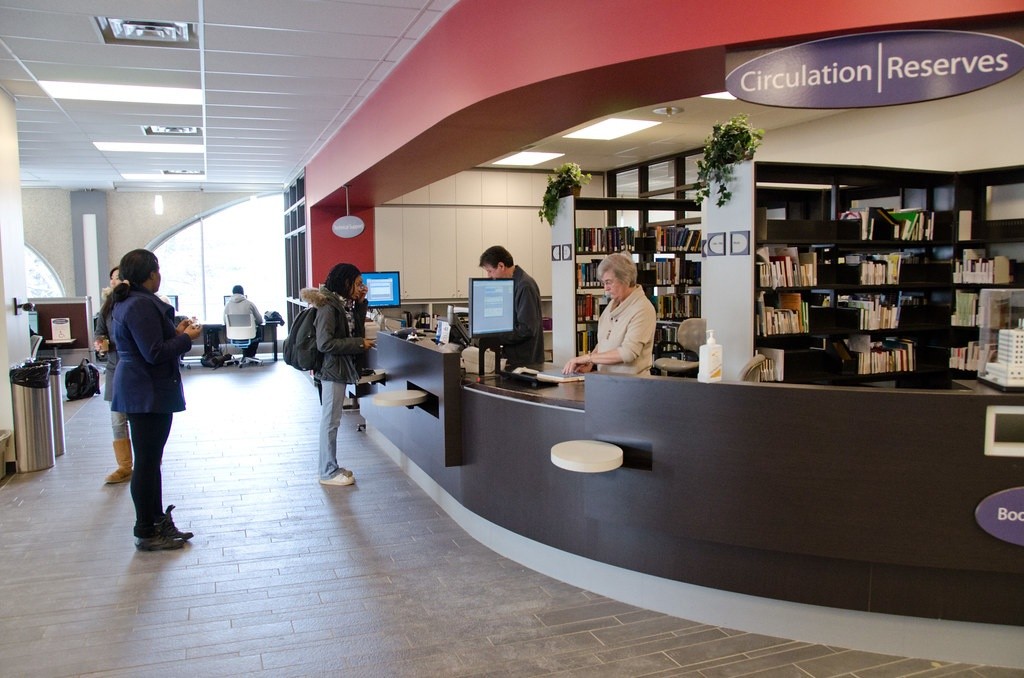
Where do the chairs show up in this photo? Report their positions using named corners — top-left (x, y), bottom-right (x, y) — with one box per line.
top-left (656, 315), bottom-right (706, 376)
top-left (221, 313), bottom-right (264, 369)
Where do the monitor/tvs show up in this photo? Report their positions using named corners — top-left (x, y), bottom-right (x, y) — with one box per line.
top-left (167, 295), bottom-right (178, 311)
top-left (468, 278), bottom-right (516, 338)
top-left (360, 271), bottom-right (401, 314)
top-left (452, 313), bottom-right (471, 345)
top-left (28, 311), bottom-right (38, 334)
top-left (224, 296), bottom-right (247, 306)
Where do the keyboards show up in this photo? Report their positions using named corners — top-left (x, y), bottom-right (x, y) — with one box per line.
top-left (500, 370), bottom-right (559, 388)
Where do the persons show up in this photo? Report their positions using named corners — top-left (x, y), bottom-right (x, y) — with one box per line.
top-left (475, 246), bottom-right (545, 364)
top-left (563, 254), bottom-right (656, 375)
top-left (94, 250), bottom-right (202, 552)
top-left (223, 285), bottom-right (262, 362)
top-left (300, 262), bottom-right (374, 485)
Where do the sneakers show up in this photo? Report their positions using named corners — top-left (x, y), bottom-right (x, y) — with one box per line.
top-left (339, 468), bottom-right (352, 477)
top-left (320, 472), bottom-right (354, 486)
top-left (135, 518), bottom-right (185, 551)
top-left (164, 505), bottom-right (194, 541)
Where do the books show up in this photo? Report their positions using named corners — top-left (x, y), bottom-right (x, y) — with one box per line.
top-left (756, 246), bottom-right (817, 287)
top-left (577, 330), bottom-right (594, 355)
top-left (757, 293), bottom-right (809, 336)
top-left (858, 338), bottom-right (914, 374)
top-left (655, 323), bottom-right (686, 361)
top-left (576, 227), bottom-right (635, 252)
top-left (757, 347), bottom-right (784, 381)
top-left (578, 294), bottom-right (599, 321)
top-left (839, 208), bottom-right (1009, 329)
top-left (654, 293), bottom-right (701, 318)
top-left (951, 340), bottom-right (998, 371)
top-left (641, 227), bottom-right (701, 252)
top-left (577, 263), bottom-right (604, 286)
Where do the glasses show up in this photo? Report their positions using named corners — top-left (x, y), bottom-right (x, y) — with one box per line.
top-left (601, 278), bottom-right (617, 287)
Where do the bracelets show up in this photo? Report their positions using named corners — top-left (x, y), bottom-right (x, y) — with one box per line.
top-left (588, 354), bottom-right (593, 363)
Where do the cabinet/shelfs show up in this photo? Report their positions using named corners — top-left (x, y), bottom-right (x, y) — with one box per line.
top-left (547, 194), bottom-right (1024, 390)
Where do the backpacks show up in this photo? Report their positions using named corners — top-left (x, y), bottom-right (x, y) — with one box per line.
top-left (281, 306), bottom-right (339, 371)
top-left (65, 358), bottom-right (101, 398)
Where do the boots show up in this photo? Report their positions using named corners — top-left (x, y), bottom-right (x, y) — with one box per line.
top-left (104, 439), bottom-right (134, 483)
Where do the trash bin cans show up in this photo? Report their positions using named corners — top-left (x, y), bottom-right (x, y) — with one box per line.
top-left (25, 357), bottom-right (66, 458)
top-left (10, 362), bottom-right (56, 474)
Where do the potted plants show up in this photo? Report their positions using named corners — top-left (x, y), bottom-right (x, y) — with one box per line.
top-left (689, 111), bottom-right (769, 208)
top-left (538, 161), bottom-right (594, 226)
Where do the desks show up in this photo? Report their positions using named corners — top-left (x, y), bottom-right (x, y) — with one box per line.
top-left (203, 320), bottom-right (281, 361)
